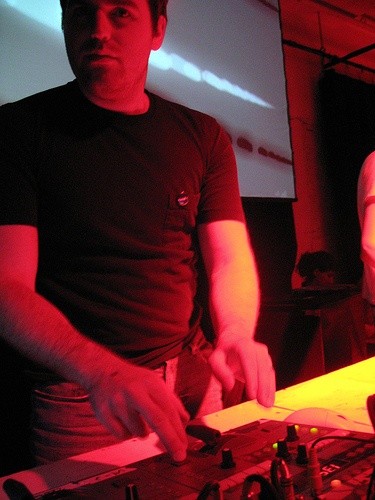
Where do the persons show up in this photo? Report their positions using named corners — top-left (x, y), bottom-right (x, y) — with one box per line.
top-left (357, 150), bottom-right (375, 357)
top-left (0, 2), bottom-right (275, 468)
